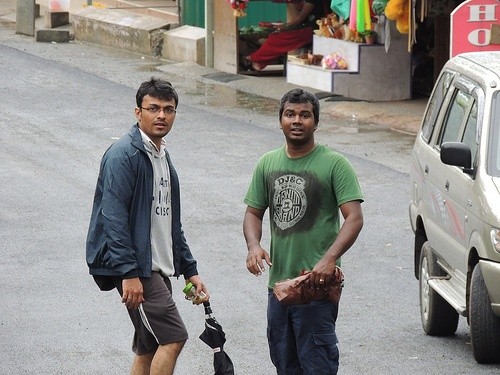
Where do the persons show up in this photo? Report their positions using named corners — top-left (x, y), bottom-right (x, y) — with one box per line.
top-left (240, 0), bottom-right (331, 70)
top-left (240, 88), bottom-right (363, 375)
top-left (85, 76), bottom-right (210, 375)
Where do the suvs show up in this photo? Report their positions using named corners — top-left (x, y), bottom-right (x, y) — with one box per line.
top-left (407, 50), bottom-right (500, 366)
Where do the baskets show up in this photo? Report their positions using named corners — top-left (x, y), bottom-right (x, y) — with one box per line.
top-left (297, 53), bottom-right (324, 64)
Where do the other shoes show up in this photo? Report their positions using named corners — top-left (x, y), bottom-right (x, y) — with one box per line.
top-left (239, 56), bottom-right (255, 71)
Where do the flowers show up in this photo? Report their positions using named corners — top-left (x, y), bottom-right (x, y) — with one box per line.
top-left (323, 54), bottom-right (346, 69)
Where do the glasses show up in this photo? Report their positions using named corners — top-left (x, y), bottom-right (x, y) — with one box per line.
top-left (141, 106), bottom-right (176, 116)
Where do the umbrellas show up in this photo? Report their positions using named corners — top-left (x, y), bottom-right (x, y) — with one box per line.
top-left (199, 299), bottom-right (235, 375)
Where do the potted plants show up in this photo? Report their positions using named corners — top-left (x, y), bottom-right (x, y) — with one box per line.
top-left (363, 30), bottom-right (379, 44)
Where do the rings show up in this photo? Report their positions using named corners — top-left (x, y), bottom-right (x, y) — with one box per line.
top-left (320, 279), bottom-right (325, 281)
